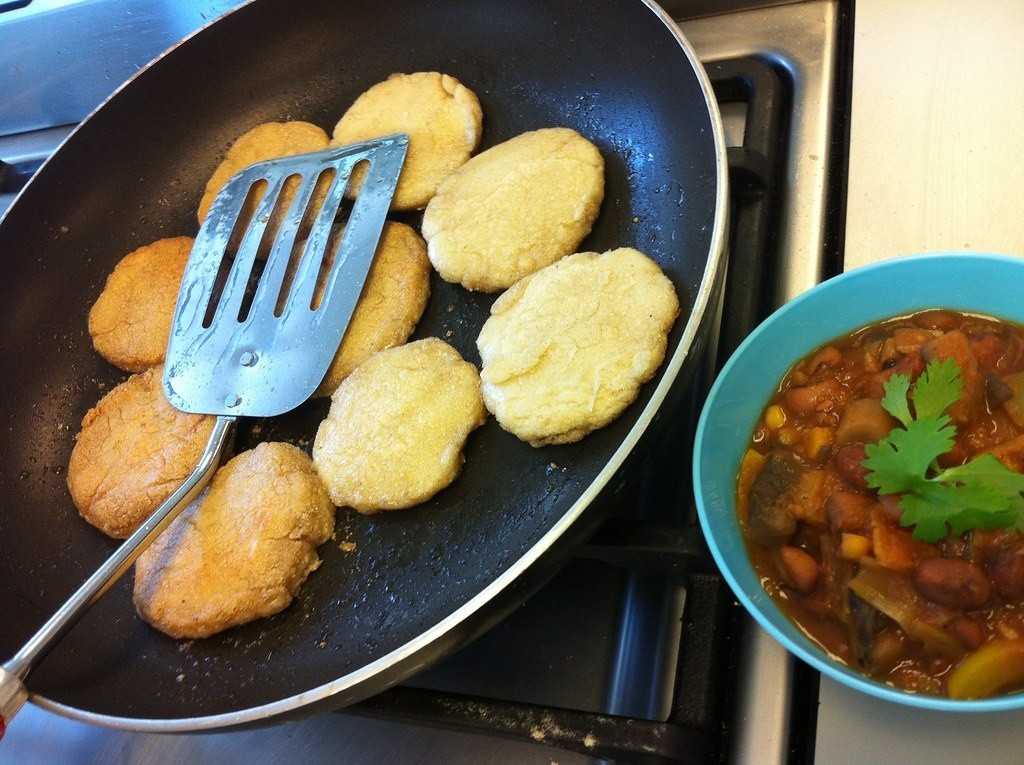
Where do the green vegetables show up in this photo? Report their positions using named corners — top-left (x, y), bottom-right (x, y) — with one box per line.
top-left (860, 359), bottom-right (1024, 546)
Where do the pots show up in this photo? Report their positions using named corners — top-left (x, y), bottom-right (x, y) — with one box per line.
top-left (1, 0), bottom-right (730, 730)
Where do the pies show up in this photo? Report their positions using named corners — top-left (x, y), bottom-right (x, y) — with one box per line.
top-left (65, 70), bottom-right (682, 640)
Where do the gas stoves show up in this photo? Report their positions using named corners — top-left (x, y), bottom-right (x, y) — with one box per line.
top-left (6, 52), bottom-right (791, 763)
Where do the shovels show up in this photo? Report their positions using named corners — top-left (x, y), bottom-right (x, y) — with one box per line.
top-left (0, 132), bottom-right (413, 736)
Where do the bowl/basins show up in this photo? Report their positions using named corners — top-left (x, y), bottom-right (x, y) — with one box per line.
top-left (686, 252), bottom-right (1023, 715)
